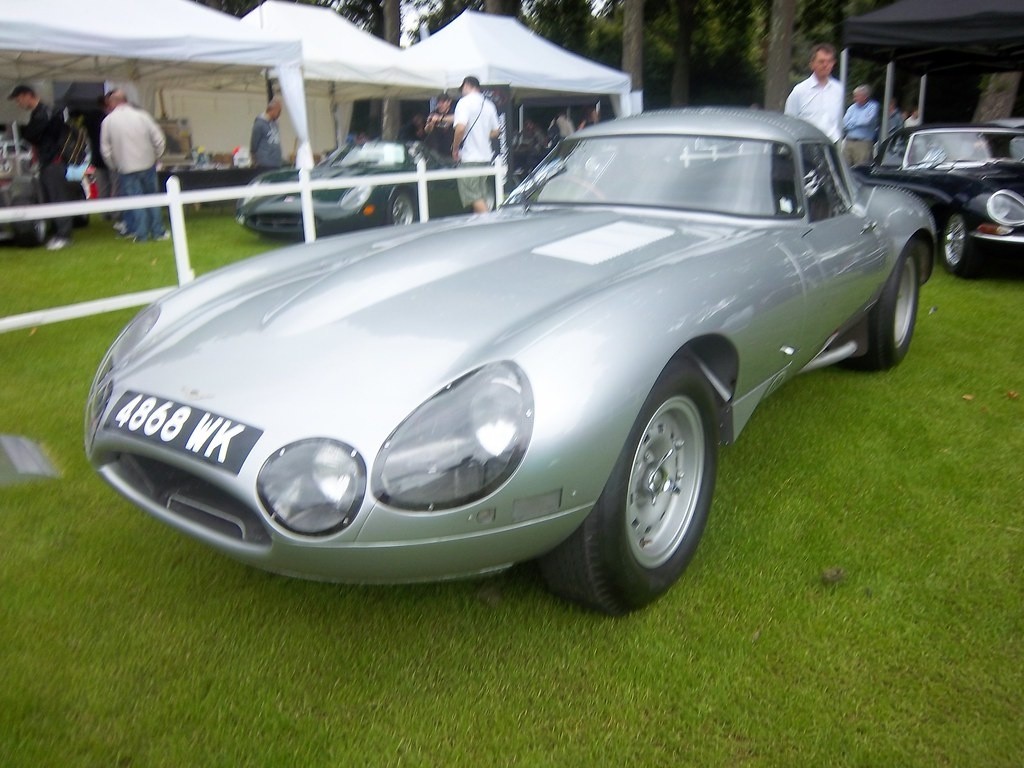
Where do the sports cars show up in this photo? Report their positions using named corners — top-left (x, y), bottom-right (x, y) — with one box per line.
top-left (86, 105), bottom-right (941, 615)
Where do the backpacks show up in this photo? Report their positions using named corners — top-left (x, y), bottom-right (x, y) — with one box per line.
top-left (55, 120), bottom-right (90, 166)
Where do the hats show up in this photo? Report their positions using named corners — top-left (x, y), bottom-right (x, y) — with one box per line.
top-left (7, 86), bottom-right (35, 101)
top-left (458, 76), bottom-right (482, 91)
top-left (436, 93), bottom-right (449, 102)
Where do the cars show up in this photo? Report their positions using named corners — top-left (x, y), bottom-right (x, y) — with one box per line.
top-left (844, 121), bottom-right (1024, 277)
top-left (236, 137), bottom-right (472, 241)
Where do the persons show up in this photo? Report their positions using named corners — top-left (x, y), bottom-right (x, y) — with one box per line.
top-left (452, 76), bottom-right (500, 214)
top-left (85, 92), bottom-right (124, 221)
top-left (841, 84), bottom-right (879, 168)
top-left (6, 85), bottom-right (72, 251)
top-left (248, 99), bottom-right (284, 175)
top-left (885, 99), bottom-right (920, 135)
top-left (783, 43), bottom-right (841, 145)
top-left (423, 92), bottom-right (454, 159)
top-left (519, 107), bottom-right (598, 174)
top-left (99, 90), bottom-right (171, 242)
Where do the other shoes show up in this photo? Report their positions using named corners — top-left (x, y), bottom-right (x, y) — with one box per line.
top-left (113, 221), bottom-right (127, 231)
top-left (156, 230), bottom-right (171, 242)
top-left (48, 238), bottom-right (68, 251)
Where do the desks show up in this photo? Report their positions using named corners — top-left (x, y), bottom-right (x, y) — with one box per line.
top-left (156, 165), bottom-right (279, 220)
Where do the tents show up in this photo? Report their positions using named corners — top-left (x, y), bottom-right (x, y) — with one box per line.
top-left (834, 0), bottom-right (1024, 140)
top-left (0, 0), bottom-right (316, 185)
top-left (162, 0), bottom-right (632, 144)
